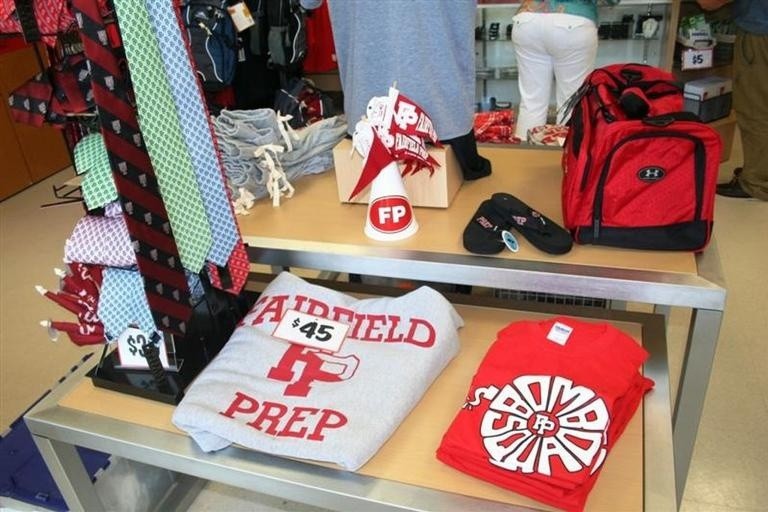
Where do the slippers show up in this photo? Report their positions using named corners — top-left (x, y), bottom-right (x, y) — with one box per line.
top-left (490, 193), bottom-right (573, 255)
top-left (463, 199), bottom-right (510, 253)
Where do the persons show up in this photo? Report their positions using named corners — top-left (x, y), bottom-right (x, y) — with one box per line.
top-left (512, 0), bottom-right (620, 141)
top-left (698, 0), bottom-right (768, 201)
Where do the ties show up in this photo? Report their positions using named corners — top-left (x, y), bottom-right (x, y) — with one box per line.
top-left (7, 50), bottom-right (95, 131)
top-left (66, 0), bottom-right (250, 342)
top-left (0, 1), bottom-right (76, 48)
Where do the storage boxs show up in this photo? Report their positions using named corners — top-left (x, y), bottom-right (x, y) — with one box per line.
top-left (333, 141), bottom-right (462, 208)
top-left (709, 115), bottom-right (736, 161)
top-left (685, 76), bottom-right (733, 123)
top-left (0, 351), bottom-right (117, 512)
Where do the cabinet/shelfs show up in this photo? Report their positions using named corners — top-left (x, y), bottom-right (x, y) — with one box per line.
top-left (477, 0), bottom-right (673, 97)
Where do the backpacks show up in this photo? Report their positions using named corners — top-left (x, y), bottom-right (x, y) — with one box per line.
top-left (231, 0), bottom-right (335, 129)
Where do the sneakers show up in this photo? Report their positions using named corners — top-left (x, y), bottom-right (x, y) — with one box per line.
top-left (716, 167), bottom-right (751, 198)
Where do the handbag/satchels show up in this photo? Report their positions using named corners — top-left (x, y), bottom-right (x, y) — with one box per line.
top-left (561, 63), bottom-right (724, 253)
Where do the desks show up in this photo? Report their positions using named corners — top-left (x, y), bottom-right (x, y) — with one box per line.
top-left (236, 142), bottom-right (728, 512)
top-left (23, 270), bottom-right (679, 512)
